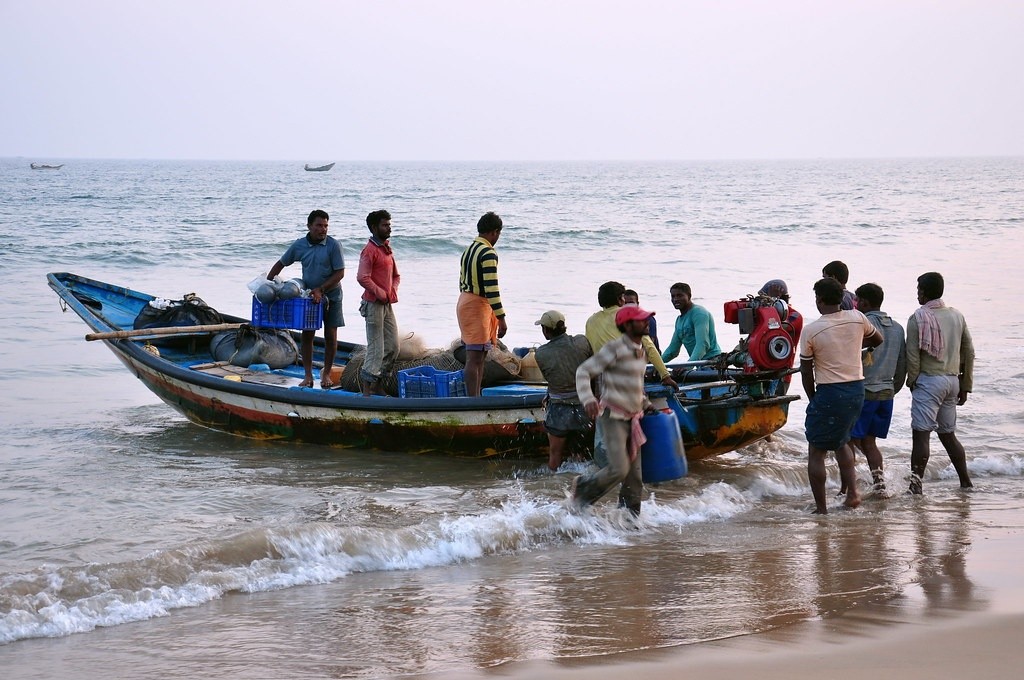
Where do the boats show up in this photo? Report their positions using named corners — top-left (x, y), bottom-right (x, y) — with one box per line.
top-left (47, 270), bottom-right (800, 461)
top-left (30, 162), bottom-right (66, 171)
top-left (304, 163), bottom-right (335, 171)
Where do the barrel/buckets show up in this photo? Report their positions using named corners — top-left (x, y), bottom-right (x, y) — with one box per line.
top-left (640, 408), bottom-right (688, 483)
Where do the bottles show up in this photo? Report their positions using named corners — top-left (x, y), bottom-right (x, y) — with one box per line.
top-left (521, 349), bottom-right (544, 382)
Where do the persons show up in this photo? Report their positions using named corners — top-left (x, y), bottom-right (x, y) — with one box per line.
top-left (625, 289), bottom-right (661, 355)
top-left (535, 310), bottom-right (601, 472)
top-left (356, 210), bottom-right (400, 396)
top-left (759, 279), bottom-right (803, 441)
top-left (799, 278), bottom-right (883, 514)
top-left (836, 283), bottom-right (906, 495)
top-left (905, 272), bottom-right (975, 493)
top-left (571, 306), bottom-right (655, 518)
top-left (652, 283), bottom-right (720, 376)
top-left (822, 261), bottom-right (857, 310)
top-left (585, 281), bottom-right (679, 470)
top-left (457, 212), bottom-right (507, 396)
top-left (267, 210), bottom-right (345, 387)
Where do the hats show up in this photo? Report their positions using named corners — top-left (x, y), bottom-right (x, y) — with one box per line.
top-left (615, 306), bottom-right (655, 327)
top-left (535, 311), bottom-right (565, 329)
top-left (758, 280), bottom-right (788, 298)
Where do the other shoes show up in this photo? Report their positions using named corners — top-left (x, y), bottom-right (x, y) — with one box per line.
top-left (572, 475), bottom-right (584, 500)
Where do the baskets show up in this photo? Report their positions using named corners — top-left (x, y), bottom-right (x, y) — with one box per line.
top-left (251, 295), bottom-right (324, 330)
top-left (398, 366), bottom-right (466, 398)
